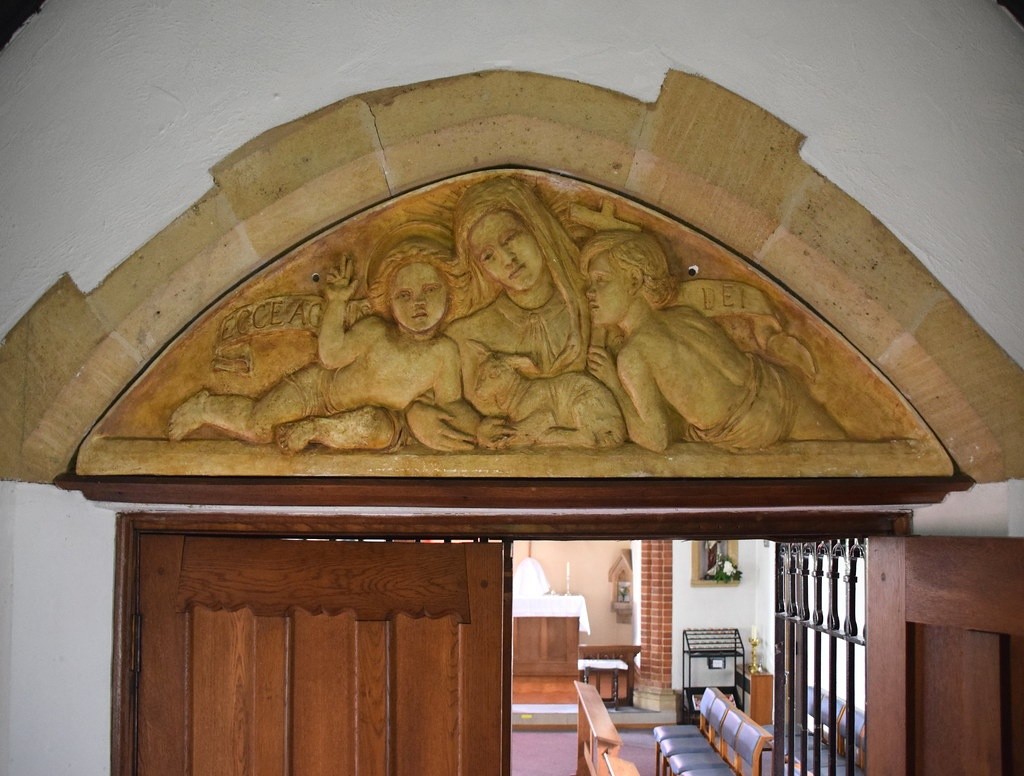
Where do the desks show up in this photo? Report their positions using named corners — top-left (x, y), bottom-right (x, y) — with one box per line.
top-left (512, 594), bottom-right (590, 676)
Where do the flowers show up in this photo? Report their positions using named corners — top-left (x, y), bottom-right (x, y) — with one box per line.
top-left (706, 553), bottom-right (742, 584)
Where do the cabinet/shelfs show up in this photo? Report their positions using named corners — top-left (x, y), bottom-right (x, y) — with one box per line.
top-left (737, 664), bottom-right (773, 725)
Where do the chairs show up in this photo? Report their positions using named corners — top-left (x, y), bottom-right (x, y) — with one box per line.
top-left (652, 687), bottom-right (814, 776)
top-left (807, 685), bottom-right (866, 770)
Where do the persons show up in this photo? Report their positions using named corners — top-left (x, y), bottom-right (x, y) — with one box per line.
top-left (580, 230), bottom-right (851, 454)
top-left (405, 175), bottom-right (630, 454)
top-left (167, 219), bottom-right (516, 459)
